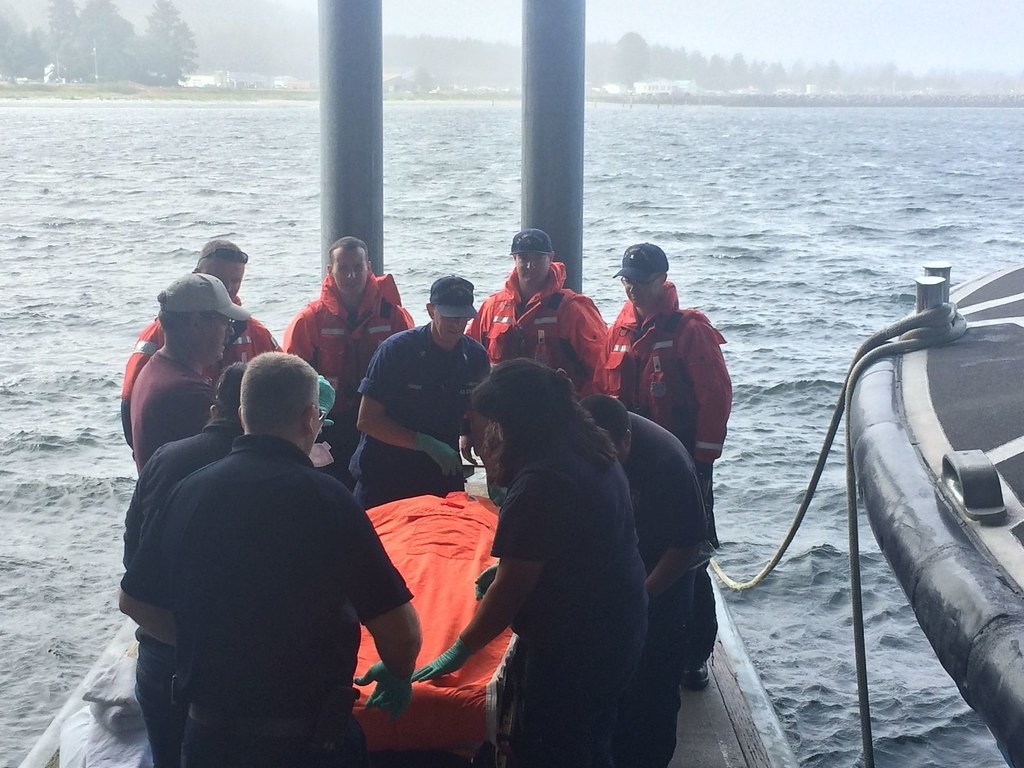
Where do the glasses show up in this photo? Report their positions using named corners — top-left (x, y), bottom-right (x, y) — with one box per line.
top-left (196, 248), bottom-right (249, 268)
top-left (621, 276), bottom-right (661, 287)
top-left (302, 405), bottom-right (328, 422)
top-left (432, 306), bottom-right (472, 322)
top-left (198, 311), bottom-right (235, 323)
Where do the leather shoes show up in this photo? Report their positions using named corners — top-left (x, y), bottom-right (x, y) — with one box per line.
top-left (682, 661), bottom-right (709, 689)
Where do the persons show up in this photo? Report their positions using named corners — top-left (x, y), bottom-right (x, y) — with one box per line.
top-left (120, 228), bottom-right (732, 768)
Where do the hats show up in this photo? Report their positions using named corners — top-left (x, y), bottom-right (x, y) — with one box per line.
top-left (613, 242), bottom-right (668, 281)
top-left (510, 228), bottom-right (552, 255)
top-left (317, 375), bottom-right (336, 433)
top-left (159, 273), bottom-right (251, 321)
top-left (430, 275), bottom-right (478, 319)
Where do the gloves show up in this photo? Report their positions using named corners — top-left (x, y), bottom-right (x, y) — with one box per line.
top-left (414, 432), bottom-right (463, 477)
top-left (355, 660), bottom-right (413, 721)
top-left (411, 637), bottom-right (471, 682)
top-left (474, 564), bottom-right (499, 600)
top-left (488, 479), bottom-right (509, 509)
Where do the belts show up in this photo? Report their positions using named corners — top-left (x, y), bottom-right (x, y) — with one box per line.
top-left (187, 678), bottom-right (354, 740)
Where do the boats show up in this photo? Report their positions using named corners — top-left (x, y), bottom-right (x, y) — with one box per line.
top-left (846, 260), bottom-right (1024, 768)
